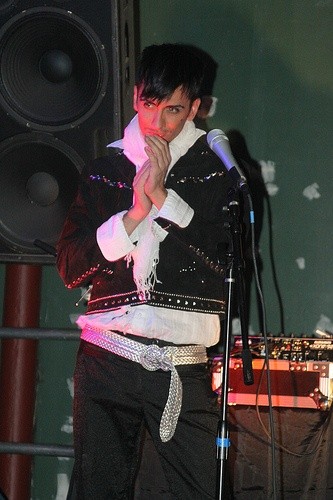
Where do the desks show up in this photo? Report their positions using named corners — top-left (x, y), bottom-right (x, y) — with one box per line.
top-left (217, 392), bottom-right (333, 500)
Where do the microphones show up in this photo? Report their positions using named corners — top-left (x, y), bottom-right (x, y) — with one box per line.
top-left (206, 128), bottom-right (251, 194)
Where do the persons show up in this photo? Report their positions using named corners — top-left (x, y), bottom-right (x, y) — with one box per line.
top-left (55, 44), bottom-right (246, 500)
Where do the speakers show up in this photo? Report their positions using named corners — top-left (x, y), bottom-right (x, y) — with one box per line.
top-left (0, 0), bottom-right (141, 266)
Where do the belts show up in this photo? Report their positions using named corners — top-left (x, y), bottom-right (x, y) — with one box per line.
top-left (78, 322), bottom-right (208, 445)
